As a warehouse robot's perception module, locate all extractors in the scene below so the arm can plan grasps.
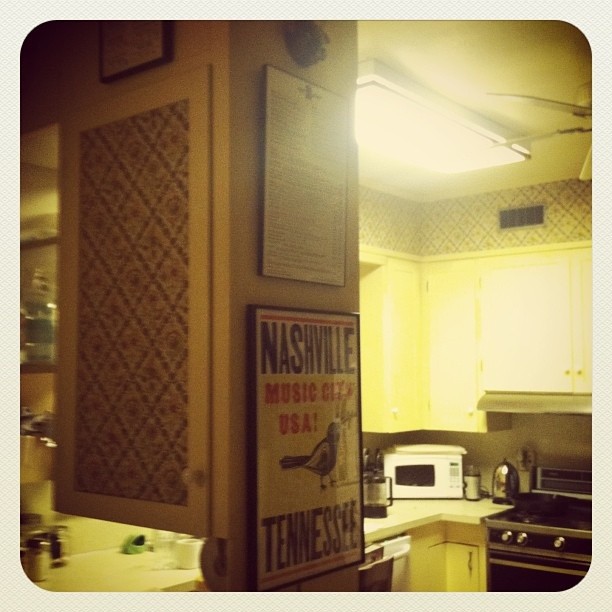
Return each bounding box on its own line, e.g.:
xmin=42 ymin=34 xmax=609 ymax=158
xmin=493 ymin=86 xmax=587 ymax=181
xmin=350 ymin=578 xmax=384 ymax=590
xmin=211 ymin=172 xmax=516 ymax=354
xmin=475 ymin=390 xmax=592 ymax=415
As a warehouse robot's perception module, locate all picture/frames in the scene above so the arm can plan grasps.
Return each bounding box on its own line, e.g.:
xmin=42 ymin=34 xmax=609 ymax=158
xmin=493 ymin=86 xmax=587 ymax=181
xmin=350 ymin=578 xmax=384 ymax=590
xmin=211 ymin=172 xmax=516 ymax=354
xmin=247 ymin=305 xmax=364 ymax=588
xmin=99 ymin=20 xmax=175 ymax=82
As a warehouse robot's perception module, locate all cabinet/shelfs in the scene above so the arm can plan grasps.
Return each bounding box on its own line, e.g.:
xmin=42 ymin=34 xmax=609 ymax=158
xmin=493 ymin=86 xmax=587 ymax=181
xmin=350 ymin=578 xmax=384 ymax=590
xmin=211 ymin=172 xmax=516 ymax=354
xmin=420 ymin=242 xmax=512 ymax=433
xmin=476 ymin=243 xmax=591 ymax=417
xmin=409 ymin=528 xmax=486 ymax=592
xmin=360 ymin=242 xmax=420 ymax=428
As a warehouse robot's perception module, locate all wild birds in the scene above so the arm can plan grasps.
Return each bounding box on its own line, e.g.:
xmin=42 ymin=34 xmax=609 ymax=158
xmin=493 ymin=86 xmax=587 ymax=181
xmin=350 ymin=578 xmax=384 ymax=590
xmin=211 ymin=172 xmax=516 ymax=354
xmin=280 ymin=422 xmax=343 ymax=490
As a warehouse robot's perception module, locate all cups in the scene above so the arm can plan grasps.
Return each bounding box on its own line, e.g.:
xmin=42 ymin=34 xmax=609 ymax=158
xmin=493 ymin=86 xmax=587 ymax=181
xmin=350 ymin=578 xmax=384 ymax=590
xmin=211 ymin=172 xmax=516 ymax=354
xmin=465 ymin=465 xmax=482 ymax=502
xmin=175 ymin=539 xmax=202 ymax=569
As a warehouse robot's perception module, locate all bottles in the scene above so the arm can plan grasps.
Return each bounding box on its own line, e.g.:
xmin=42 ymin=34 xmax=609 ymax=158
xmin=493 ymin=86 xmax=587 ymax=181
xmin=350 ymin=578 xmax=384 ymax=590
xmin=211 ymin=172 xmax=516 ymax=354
xmin=50 ymin=526 xmax=71 ymax=570
xmin=26 ymin=537 xmax=51 ymax=582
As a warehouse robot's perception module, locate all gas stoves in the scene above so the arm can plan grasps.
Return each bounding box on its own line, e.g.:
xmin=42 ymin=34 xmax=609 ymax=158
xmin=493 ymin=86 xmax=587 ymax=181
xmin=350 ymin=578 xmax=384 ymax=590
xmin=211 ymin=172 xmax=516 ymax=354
xmin=482 ymin=505 xmax=593 ymax=534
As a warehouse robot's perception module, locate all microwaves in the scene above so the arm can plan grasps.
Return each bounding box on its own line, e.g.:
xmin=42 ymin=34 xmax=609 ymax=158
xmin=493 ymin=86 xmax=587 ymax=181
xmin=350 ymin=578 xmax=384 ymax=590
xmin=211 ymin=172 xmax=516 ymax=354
xmin=383 ymin=452 xmax=462 ymax=501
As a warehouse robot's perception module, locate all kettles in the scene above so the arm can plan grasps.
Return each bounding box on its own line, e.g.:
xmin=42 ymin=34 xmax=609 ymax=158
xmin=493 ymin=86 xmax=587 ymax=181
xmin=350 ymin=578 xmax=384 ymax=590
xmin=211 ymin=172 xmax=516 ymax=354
xmin=363 ymin=467 xmax=392 ymax=518
xmin=491 ymin=459 xmax=519 ymax=505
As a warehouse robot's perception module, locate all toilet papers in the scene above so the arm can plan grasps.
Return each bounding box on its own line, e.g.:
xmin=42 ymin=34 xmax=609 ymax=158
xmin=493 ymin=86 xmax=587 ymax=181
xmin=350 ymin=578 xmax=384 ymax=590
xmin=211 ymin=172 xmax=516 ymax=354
xmin=174 ymin=536 xmax=203 ymax=572
